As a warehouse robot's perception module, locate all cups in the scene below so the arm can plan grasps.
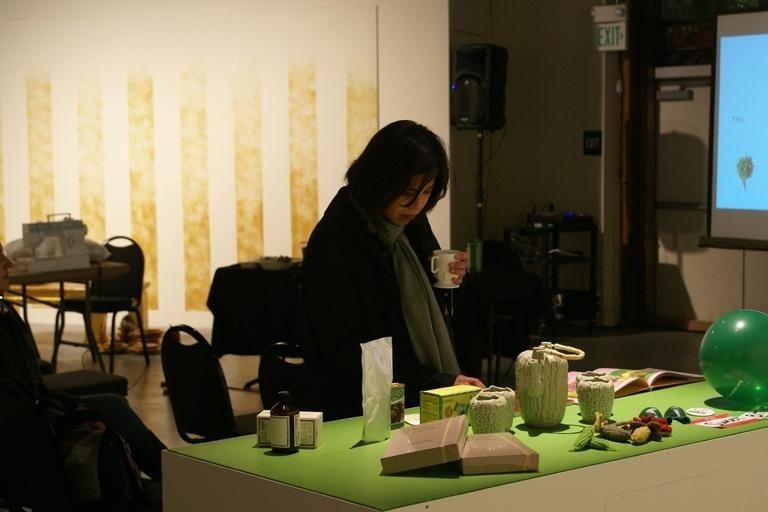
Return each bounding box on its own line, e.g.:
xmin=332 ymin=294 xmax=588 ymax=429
xmin=575 ymin=370 xmax=608 ymax=393
xmin=580 ymin=379 xmax=614 ymax=424
xmin=479 ymin=384 xmax=515 ymax=432
xmin=429 ymin=249 xmax=463 ymax=289
xmin=468 ymin=393 xmax=507 ymax=434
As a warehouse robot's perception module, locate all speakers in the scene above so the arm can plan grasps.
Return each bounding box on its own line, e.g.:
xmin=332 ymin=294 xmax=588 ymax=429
xmin=452 ymin=40 xmax=508 ymax=131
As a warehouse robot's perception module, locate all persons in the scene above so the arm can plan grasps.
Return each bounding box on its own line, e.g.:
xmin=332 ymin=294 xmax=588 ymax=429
xmin=1 ymin=246 xmax=165 ymax=510
xmin=299 ymin=119 xmax=486 ymax=416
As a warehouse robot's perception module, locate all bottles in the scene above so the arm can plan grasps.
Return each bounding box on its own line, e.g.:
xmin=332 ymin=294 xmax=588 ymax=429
xmin=466 ymin=239 xmax=483 ymax=272
xmin=271 ymin=389 xmax=301 ymax=455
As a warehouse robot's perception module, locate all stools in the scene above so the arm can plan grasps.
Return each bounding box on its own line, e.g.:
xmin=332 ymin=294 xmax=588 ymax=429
xmin=42 ymin=368 xmax=128 ymax=398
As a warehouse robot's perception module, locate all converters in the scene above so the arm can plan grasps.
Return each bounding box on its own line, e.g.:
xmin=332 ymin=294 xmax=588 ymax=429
xmin=548 ymin=202 xmax=554 ymax=212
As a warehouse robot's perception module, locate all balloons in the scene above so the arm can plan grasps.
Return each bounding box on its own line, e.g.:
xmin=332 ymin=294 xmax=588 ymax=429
xmin=699 ymin=308 xmax=768 ymax=408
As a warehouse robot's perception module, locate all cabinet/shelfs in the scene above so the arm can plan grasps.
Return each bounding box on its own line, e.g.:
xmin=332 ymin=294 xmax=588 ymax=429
xmin=508 ymin=215 xmax=597 ymax=338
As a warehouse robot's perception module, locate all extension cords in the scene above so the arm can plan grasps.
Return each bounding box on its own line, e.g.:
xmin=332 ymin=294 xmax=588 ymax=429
xmin=535 ymin=208 xmax=560 ymax=218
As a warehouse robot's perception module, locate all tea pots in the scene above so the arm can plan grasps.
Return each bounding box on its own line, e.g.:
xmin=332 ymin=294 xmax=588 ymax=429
xmin=515 ymin=342 xmax=586 ymax=428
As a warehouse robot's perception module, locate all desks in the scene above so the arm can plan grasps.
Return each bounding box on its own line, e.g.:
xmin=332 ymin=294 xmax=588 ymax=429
xmin=162 ymin=372 xmax=768 ymax=512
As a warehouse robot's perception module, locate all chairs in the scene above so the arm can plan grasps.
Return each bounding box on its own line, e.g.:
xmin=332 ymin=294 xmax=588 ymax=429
xmin=259 ymin=341 xmax=313 ymax=413
xmin=161 ymin=323 xmax=264 ymax=443
xmin=52 ymin=236 xmax=150 ymax=373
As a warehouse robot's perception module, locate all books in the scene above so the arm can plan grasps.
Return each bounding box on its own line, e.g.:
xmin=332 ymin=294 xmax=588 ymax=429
xmin=460 ymin=432 xmax=539 ymax=475
xmin=380 ymin=414 xmax=468 ymax=474
xmin=567 ymin=367 xmax=704 ymax=405
